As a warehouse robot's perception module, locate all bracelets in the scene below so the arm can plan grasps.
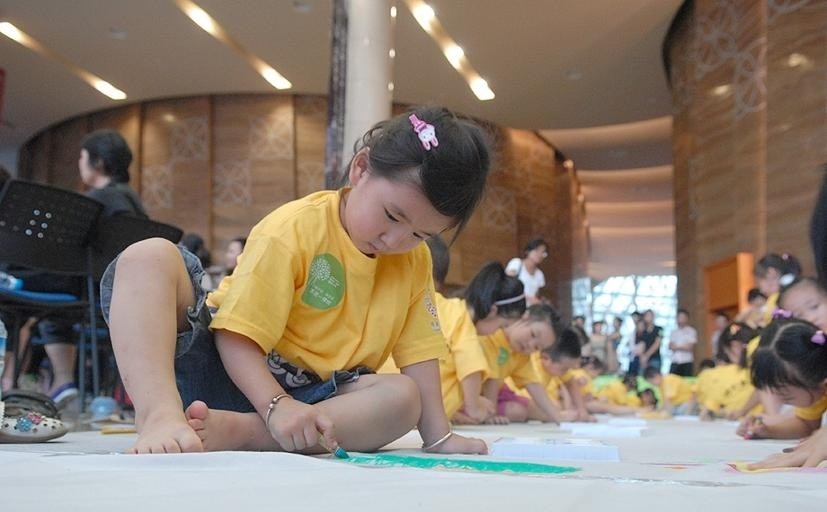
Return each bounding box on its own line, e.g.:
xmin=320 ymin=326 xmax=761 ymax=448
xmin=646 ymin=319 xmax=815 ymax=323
xmin=265 ymin=392 xmax=292 ymax=425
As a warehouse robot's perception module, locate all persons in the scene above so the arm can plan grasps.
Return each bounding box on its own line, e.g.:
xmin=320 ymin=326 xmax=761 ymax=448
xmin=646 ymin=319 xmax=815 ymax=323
xmin=99 ymin=103 xmax=492 ymax=456
xmin=180 ymin=231 xmax=244 ymax=291
xmin=691 ymin=168 xmax=827 ymax=471
xmin=36 ymin=128 xmax=151 ymax=419
xmin=380 ymin=231 xmax=698 ymax=425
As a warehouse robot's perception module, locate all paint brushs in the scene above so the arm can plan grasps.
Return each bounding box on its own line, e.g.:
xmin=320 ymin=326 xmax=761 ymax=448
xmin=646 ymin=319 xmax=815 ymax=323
xmin=743 ymin=419 xmax=762 ymax=439
xmin=318 ymin=434 xmax=350 ymax=458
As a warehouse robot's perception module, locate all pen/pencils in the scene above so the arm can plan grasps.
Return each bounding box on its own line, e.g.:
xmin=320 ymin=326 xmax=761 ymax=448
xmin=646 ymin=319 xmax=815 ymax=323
xmin=782 ymin=447 xmax=796 ymax=453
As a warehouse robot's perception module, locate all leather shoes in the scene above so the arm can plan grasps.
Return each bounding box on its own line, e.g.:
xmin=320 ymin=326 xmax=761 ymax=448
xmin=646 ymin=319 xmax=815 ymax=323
xmin=1 ymin=399 xmax=70 ymax=444
